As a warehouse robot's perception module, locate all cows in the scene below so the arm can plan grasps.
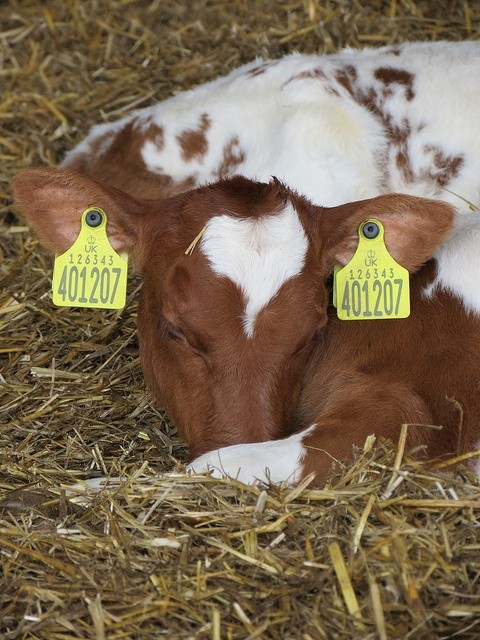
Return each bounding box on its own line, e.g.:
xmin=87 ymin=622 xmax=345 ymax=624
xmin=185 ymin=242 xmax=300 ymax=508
xmin=10 ymin=40 xmax=479 ymax=492
